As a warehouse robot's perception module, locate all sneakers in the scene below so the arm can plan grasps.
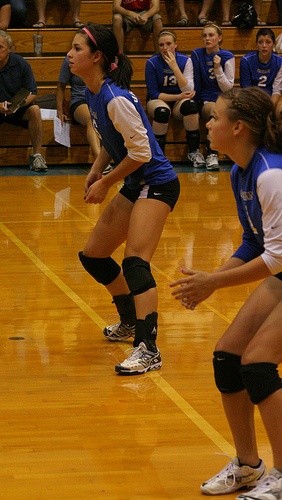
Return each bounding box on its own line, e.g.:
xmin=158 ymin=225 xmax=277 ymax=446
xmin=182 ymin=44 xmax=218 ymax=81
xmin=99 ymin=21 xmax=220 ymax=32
xmin=102 ymin=164 xmax=113 ymax=175
xmin=102 ymin=321 xmax=135 ymax=341
xmin=199 ymin=457 xmax=266 ymax=496
xmin=29 ymin=153 xmax=48 ymax=172
xmin=114 ymin=341 xmax=162 ymax=374
xmin=188 ymin=149 xmax=206 ymax=168
xmin=237 ymin=467 xmax=282 ymax=500
xmin=205 ymin=153 xmax=221 ymax=172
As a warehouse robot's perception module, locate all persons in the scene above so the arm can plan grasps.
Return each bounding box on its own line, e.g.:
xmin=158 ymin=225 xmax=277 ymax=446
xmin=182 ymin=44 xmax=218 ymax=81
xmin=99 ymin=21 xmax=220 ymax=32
xmin=113 ymin=0 xmax=164 ymax=55
xmin=191 ymin=23 xmax=235 ymax=170
xmin=173 ymin=0 xmax=267 ymax=26
xmin=0 ymin=0 xmax=85 ymax=28
xmin=170 ymin=85 xmax=282 ymax=500
xmin=145 ymin=28 xmax=207 ymax=169
xmin=0 ymin=30 xmax=49 ymax=172
xmin=56 ymin=55 xmax=113 ymax=175
xmin=67 ymin=23 xmax=180 ymax=375
xmin=239 ymin=29 xmax=282 ymax=98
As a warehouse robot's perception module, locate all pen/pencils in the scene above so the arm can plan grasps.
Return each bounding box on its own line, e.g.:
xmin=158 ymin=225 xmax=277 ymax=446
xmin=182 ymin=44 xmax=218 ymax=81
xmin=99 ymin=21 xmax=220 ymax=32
xmin=5 ymin=101 xmax=7 ymax=116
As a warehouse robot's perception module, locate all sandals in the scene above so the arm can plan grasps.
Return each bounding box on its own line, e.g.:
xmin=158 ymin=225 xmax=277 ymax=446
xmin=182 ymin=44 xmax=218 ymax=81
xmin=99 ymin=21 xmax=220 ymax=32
xmin=256 ymin=19 xmax=267 ymax=26
xmin=195 ymin=17 xmax=215 ymax=27
xmin=175 ymin=15 xmax=189 ymax=27
xmin=221 ymin=21 xmax=232 ymax=27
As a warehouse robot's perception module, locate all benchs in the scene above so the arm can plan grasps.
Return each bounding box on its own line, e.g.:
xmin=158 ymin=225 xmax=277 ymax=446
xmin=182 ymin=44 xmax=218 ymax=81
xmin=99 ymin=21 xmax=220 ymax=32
xmin=1 ymin=0 xmax=282 ymax=167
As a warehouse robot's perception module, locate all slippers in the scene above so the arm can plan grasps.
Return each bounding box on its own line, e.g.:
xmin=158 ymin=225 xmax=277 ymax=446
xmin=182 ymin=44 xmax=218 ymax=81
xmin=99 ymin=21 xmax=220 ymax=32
xmin=33 ymin=22 xmax=47 ymax=28
xmin=68 ymin=21 xmax=85 ymax=28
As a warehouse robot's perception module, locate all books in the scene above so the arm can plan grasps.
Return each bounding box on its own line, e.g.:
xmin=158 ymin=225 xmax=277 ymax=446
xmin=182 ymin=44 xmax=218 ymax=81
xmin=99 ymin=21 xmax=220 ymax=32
xmin=7 ymin=88 xmax=30 ymax=113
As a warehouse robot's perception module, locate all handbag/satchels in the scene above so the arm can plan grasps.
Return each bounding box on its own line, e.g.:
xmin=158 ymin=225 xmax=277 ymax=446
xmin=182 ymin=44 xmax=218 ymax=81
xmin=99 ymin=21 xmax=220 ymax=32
xmin=230 ymin=1 xmax=257 ymax=30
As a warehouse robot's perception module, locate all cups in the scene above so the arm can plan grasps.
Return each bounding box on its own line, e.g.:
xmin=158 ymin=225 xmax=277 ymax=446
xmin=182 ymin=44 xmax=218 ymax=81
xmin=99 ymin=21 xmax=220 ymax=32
xmin=33 ymin=34 xmax=44 ymax=56
